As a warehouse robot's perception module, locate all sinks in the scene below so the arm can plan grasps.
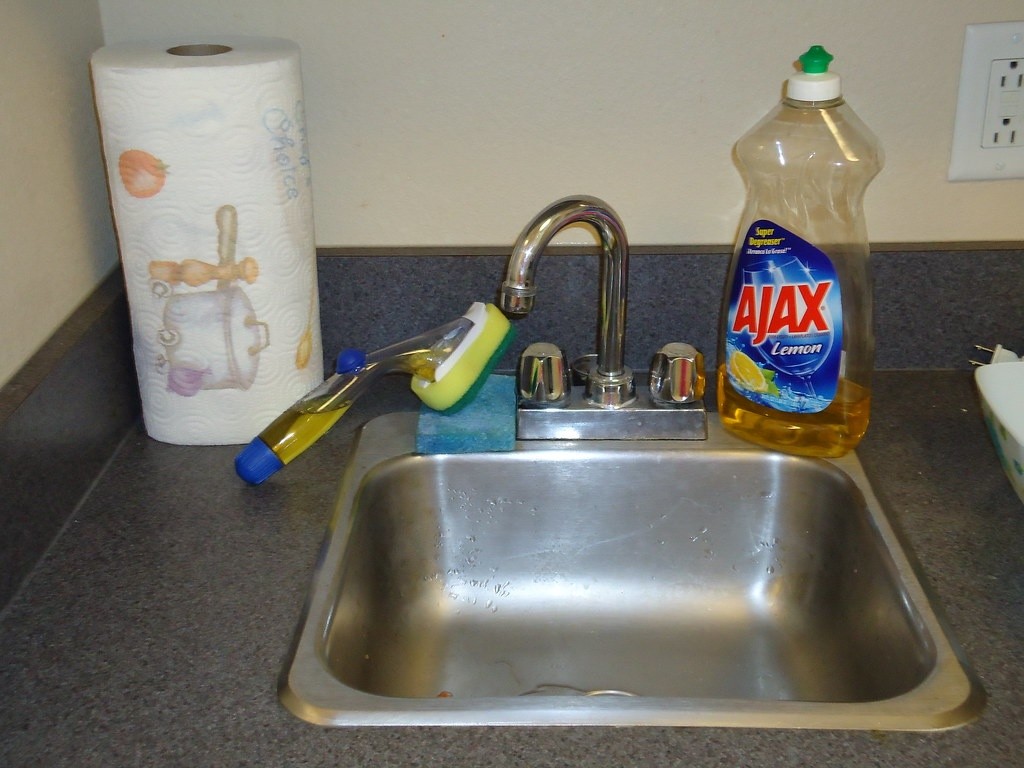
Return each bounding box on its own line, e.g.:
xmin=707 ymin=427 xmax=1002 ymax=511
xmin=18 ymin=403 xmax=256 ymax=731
xmin=277 ymin=407 xmax=989 ymax=733
xmin=499 ymin=192 xmax=709 ymax=441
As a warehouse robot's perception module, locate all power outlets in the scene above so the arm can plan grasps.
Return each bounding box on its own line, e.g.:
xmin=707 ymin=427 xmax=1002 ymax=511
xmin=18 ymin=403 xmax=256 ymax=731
xmin=948 ymin=20 xmax=1024 ymax=182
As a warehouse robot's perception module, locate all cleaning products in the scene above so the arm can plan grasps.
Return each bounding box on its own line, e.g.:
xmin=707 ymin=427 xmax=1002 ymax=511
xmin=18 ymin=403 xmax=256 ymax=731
xmin=715 ymin=42 xmax=888 ymax=460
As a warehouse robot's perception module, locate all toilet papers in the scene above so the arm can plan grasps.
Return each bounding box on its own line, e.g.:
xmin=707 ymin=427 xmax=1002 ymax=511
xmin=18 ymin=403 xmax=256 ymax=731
xmin=89 ymin=32 xmax=327 ymax=447
